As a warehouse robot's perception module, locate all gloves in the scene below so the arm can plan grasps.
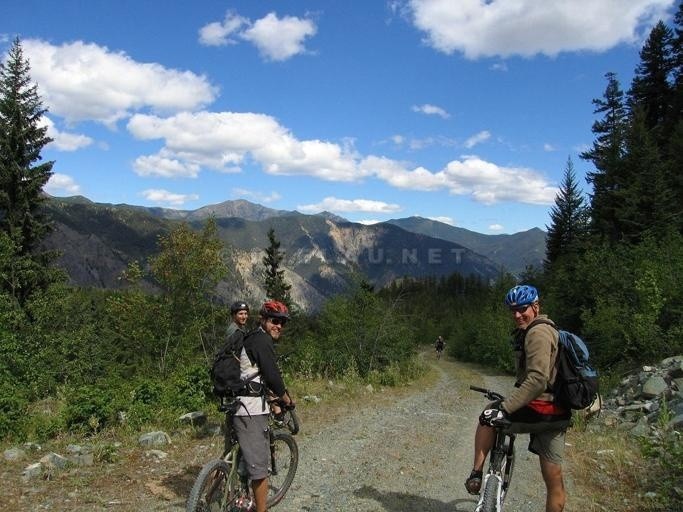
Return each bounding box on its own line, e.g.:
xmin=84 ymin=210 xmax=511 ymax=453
xmin=479 ymin=408 xmax=505 ymax=426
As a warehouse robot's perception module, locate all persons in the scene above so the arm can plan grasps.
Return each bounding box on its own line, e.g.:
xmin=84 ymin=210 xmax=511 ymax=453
xmin=224 ymin=301 xmax=249 ymax=344
xmin=434 ymin=336 xmax=446 ymax=360
xmin=232 ymin=301 xmax=296 ymax=512
xmin=466 ymin=285 xmax=572 ymax=511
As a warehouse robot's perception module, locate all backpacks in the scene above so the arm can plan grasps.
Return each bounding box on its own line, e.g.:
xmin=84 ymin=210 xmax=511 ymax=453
xmin=212 ymin=328 xmax=268 ymax=397
xmin=521 ymin=319 xmax=597 ymax=409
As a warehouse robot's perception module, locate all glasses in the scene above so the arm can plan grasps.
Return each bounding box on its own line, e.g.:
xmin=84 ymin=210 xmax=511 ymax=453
xmin=268 ymin=318 xmax=286 ymax=326
xmin=510 ymin=305 xmax=529 ymax=313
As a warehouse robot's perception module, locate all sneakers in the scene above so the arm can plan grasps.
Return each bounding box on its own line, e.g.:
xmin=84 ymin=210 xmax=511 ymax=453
xmin=467 ymin=469 xmax=482 ymax=493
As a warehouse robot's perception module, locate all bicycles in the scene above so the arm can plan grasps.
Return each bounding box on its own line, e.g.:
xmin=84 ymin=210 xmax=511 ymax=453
xmin=469 ymin=384 xmax=516 ymax=512
xmin=186 ymin=396 xmax=299 ymax=511
xmin=434 ymin=344 xmax=443 ymax=360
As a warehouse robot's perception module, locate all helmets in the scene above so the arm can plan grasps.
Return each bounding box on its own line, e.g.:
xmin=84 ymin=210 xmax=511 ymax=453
xmin=260 ymin=300 xmax=289 ymax=320
xmin=230 ymin=301 xmax=249 ymax=313
xmin=504 ymin=285 xmax=540 ymax=309
